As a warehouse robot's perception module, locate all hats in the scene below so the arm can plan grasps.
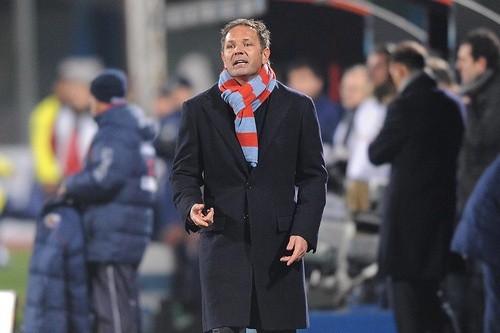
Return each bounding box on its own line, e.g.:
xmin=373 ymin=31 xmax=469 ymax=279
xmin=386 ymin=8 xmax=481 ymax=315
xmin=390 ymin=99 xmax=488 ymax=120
xmin=89 ymin=72 xmax=128 ymax=104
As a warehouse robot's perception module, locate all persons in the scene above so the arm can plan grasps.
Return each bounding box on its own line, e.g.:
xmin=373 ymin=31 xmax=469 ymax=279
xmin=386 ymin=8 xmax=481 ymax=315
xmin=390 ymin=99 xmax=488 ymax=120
xmin=285 ymin=28 xmax=500 ymax=333
xmin=170 ymin=19 xmax=329 ymax=333
xmin=28 ymin=56 xmax=202 ymax=333
